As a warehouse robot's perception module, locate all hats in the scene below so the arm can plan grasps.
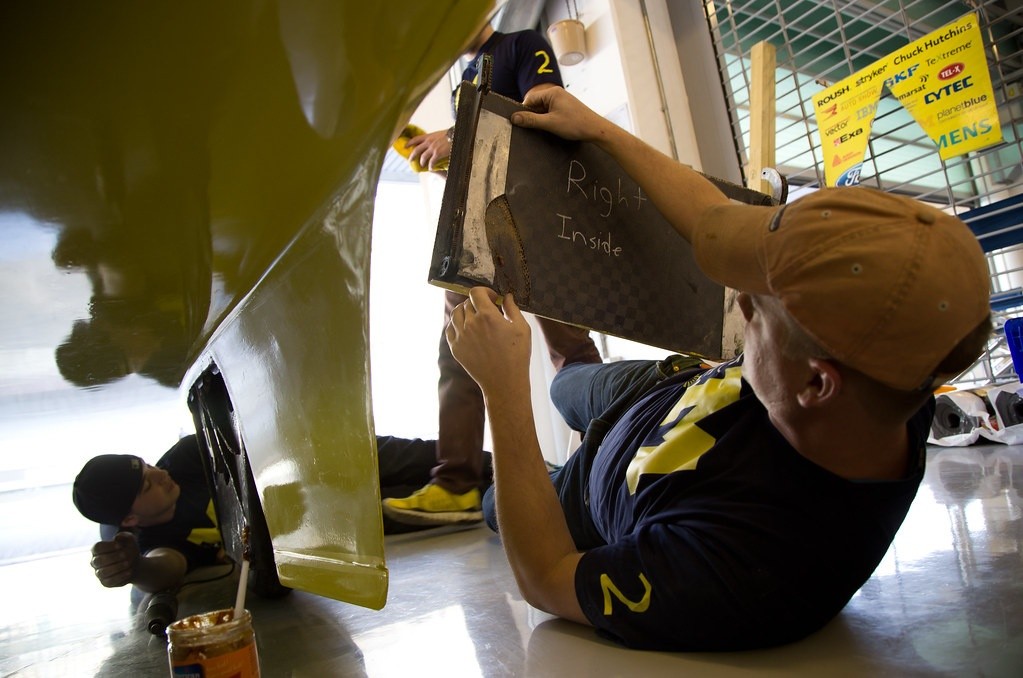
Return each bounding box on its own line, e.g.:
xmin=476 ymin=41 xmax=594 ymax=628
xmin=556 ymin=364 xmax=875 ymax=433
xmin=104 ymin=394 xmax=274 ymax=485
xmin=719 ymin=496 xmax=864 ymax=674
xmin=73 ymin=454 xmax=143 ymax=527
xmin=690 ymin=186 xmax=991 ymax=392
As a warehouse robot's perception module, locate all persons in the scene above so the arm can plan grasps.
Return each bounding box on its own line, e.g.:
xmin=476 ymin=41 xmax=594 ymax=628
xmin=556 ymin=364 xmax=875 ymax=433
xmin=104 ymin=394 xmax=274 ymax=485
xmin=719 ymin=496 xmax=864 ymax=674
xmin=445 ymin=83 xmax=993 ymax=652
xmin=381 ymin=20 xmax=603 ymax=528
xmin=70 ymin=430 xmax=565 ymax=595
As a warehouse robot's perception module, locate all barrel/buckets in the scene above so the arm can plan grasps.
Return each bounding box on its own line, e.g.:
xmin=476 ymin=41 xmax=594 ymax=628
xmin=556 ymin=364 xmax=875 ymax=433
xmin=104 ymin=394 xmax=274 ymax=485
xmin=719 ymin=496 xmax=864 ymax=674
xmin=547 ymin=20 xmax=586 ymax=66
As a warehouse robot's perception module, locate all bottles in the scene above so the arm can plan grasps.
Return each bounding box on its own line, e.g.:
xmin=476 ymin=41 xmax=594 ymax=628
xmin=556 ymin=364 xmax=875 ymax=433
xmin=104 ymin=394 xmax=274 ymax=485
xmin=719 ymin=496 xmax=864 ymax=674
xmin=165 ymin=609 xmax=261 ymax=678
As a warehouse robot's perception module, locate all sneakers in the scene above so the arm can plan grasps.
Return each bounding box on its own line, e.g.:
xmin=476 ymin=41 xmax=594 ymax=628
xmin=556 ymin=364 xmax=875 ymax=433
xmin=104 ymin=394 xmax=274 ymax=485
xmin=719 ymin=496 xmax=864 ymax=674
xmin=382 ymin=483 xmax=483 ymax=526
